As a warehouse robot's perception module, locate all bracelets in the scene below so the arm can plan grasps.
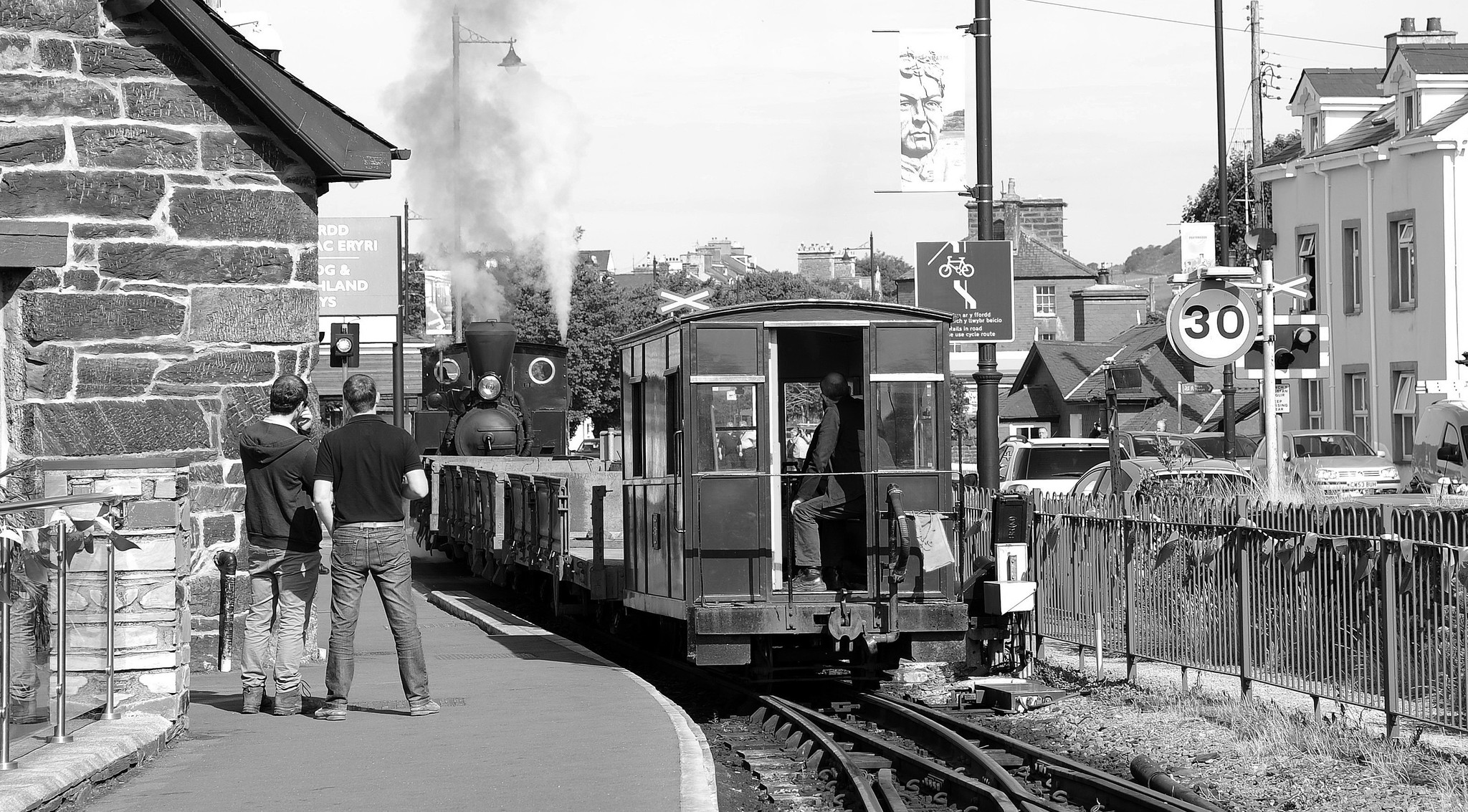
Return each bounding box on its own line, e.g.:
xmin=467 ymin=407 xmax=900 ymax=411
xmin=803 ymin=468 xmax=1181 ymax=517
xmin=739 ymin=451 xmax=742 ymax=453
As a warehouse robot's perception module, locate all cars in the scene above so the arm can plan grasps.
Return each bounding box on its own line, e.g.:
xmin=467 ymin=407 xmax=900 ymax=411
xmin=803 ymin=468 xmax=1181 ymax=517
xmin=997 ymin=430 xmax=1266 ymax=507
xmin=1250 ymin=428 xmax=1402 ymax=497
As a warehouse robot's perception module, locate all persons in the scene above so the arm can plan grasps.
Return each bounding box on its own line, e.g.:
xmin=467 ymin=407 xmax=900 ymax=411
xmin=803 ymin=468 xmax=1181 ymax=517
xmin=1038 ymin=427 xmax=1048 ymax=438
xmin=782 ymin=371 xmax=896 ymax=591
xmin=717 ymin=422 xmax=743 ymax=469
xmin=739 ymin=421 xmax=756 ymax=470
xmin=240 ymin=374 xmax=323 ymax=716
xmin=312 ymin=482 xmax=333 ymax=575
xmin=1088 ymin=423 xmax=1101 ymax=438
xmin=314 ymin=373 xmax=440 ymax=721
xmin=1325 ymin=437 xmax=1341 ymax=455
xmin=1156 ymin=421 xmax=1167 ymax=432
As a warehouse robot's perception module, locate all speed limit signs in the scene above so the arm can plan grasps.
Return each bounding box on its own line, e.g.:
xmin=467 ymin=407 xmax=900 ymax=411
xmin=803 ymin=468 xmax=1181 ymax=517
xmin=1169 ymin=283 xmax=1261 ymax=366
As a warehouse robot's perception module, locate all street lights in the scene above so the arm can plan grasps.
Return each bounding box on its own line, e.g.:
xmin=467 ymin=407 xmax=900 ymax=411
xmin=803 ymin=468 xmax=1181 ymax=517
xmin=407 ymin=290 xmax=978 ymax=692
xmin=447 ymin=5 xmax=529 ymax=347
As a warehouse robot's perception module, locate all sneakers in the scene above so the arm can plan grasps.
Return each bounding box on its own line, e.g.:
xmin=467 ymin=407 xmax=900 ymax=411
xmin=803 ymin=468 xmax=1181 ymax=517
xmin=818 ymin=565 xmax=845 ymax=590
xmin=779 ymin=568 xmax=827 ymax=591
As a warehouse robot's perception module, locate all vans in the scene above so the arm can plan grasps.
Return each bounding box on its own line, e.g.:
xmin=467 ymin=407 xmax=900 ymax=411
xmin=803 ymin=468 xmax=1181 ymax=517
xmin=1409 ymin=397 xmax=1468 ymax=498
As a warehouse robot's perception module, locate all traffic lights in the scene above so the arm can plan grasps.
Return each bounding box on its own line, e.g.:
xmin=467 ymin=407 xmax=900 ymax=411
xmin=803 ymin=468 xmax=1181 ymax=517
xmin=1247 ymin=324 xmax=1321 ymax=372
xmin=333 ymin=334 xmax=353 ymax=358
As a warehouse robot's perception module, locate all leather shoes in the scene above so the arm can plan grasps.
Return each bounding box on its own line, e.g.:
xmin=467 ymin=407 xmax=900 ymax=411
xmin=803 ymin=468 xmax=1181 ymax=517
xmin=318 ymin=563 xmax=329 ymax=574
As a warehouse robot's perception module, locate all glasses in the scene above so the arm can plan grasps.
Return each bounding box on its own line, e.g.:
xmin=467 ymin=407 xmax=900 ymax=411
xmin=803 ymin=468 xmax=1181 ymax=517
xmin=299 ymin=401 xmax=308 ymax=408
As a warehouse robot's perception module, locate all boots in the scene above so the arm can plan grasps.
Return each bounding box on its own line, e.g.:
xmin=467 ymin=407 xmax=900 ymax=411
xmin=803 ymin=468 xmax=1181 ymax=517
xmin=274 ymin=680 xmax=322 ymax=716
xmin=241 ymin=686 xmax=273 ymax=713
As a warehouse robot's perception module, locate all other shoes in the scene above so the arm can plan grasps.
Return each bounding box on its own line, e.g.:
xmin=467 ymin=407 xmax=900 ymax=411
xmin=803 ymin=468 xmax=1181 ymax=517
xmin=315 ymin=707 xmax=347 ymax=721
xmin=410 ymin=700 xmax=440 ymax=715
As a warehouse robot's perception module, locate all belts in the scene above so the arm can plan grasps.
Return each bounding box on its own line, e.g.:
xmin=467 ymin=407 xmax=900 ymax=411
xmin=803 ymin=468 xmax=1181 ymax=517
xmin=339 ymin=521 xmax=403 ymax=528
